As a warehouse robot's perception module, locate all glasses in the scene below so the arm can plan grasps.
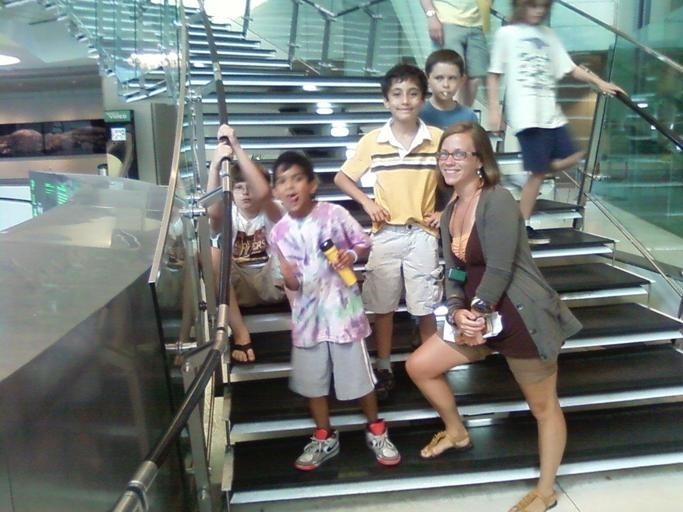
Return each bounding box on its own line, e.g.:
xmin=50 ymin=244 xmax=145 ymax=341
xmin=436 ymin=150 xmax=477 ymax=161
xmin=233 ymin=185 xmax=244 ymax=193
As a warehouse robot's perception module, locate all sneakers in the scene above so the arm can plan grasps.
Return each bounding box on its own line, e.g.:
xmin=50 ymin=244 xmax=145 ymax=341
xmin=294 ymin=430 xmax=340 ymax=470
xmin=527 ymin=225 xmax=550 ymax=245
xmin=374 ymin=369 xmax=397 ymax=401
xmin=365 ymin=418 xmax=401 ymax=465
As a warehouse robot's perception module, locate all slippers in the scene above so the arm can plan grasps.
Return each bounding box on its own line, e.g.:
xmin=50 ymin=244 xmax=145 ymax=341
xmin=231 ymin=342 xmax=257 ymax=364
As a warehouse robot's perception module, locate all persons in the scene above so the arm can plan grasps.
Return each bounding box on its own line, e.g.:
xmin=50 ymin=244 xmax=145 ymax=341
xmin=420 ymin=47 xmax=479 ymax=129
xmin=267 ymin=152 xmax=402 ymax=470
xmin=418 ymin=0 xmax=497 ymax=107
xmin=204 ymin=124 xmax=286 ymax=364
xmin=109 ymin=203 xmax=201 ymax=367
xmin=333 ymin=63 xmax=444 ymax=403
xmin=406 ymin=123 xmax=582 ymax=507
xmin=484 ymin=1 xmax=627 ymax=244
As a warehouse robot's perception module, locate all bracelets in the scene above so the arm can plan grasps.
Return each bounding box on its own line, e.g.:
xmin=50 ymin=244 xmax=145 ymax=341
xmin=345 ymin=248 xmax=359 ymax=261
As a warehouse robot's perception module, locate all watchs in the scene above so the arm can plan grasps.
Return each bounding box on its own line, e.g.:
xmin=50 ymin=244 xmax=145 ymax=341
xmin=423 ymin=8 xmax=438 ymax=17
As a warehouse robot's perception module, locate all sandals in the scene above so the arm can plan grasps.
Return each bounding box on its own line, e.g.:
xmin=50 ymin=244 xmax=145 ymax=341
xmin=420 ymin=431 xmax=474 ymax=460
xmin=508 ymin=491 xmax=558 ymax=512
